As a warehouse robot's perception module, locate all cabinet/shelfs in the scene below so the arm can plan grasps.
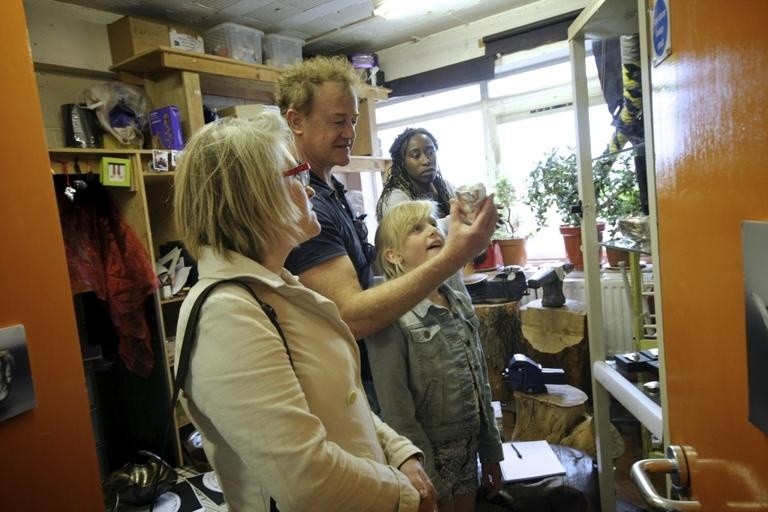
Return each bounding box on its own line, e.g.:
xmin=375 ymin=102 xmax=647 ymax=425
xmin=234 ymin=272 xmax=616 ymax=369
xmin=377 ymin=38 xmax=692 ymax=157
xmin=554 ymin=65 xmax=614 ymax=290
xmin=46 ymin=146 xmax=201 ymax=468
xmin=565 ymin=0 xmax=768 ymax=512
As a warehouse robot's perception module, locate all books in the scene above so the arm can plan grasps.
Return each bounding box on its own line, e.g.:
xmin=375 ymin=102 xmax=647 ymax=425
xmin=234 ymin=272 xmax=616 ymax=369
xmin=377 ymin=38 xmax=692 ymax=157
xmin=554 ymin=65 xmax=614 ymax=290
xmin=493 ymin=438 xmax=568 ymax=483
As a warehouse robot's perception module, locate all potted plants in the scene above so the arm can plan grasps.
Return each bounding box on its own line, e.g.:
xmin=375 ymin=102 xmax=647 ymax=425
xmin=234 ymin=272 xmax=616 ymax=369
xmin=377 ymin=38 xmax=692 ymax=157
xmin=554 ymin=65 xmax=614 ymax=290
xmin=523 ymin=147 xmax=644 ymax=273
xmin=493 ymin=177 xmax=522 ymax=266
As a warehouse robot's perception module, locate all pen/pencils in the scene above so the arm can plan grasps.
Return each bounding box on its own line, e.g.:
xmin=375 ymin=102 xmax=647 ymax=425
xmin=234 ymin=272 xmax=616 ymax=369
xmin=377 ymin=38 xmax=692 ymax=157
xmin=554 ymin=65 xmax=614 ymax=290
xmin=511 ymin=443 xmax=523 ymax=458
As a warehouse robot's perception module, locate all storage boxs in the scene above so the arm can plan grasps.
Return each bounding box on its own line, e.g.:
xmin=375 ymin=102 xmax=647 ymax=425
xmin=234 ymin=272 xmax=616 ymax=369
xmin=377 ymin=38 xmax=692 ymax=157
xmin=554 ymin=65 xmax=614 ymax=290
xmin=105 ymin=14 xmax=170 ymax=65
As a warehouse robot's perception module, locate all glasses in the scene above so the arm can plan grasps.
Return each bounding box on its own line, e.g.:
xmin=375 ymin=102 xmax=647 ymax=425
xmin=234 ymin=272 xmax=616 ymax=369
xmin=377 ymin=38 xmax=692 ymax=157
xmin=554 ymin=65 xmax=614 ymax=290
xmin=283 ymin=162 xmax=311 ymax=187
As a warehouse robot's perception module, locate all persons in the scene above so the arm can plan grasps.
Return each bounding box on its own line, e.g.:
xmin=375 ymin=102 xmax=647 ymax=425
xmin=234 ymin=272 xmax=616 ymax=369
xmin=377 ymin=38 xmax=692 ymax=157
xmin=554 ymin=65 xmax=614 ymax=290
xmin=364 ymin=195 xmax=507 ymax=510
xmin=260 ymin=53 xmax=501 ymax=385
xmin=163 ymin=107 xmax=448 ymax=512
xmin=373 ymin=126 xmax=476 ymax=300
